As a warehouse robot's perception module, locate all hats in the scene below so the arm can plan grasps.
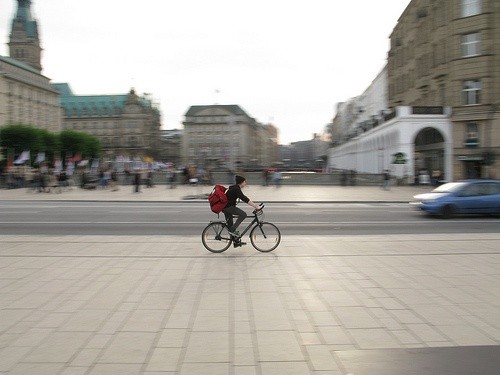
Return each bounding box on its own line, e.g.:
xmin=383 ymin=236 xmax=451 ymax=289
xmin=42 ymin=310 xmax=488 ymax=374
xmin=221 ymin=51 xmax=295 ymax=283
xmin=236 ymin=176 xmax=246 ymax=185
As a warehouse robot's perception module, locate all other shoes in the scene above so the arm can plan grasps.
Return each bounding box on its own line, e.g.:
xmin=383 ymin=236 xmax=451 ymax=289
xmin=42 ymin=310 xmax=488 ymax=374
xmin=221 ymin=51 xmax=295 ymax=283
xmin=229 ymin=230 xmax=242 ymax=239
xmin=234 ymin=241 xmax=247 ymax=248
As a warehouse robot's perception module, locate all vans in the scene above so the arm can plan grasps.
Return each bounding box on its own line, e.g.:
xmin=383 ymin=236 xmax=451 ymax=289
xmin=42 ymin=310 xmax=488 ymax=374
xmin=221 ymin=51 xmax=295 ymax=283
xmin=409 ymin=179 xmax=500 ymax=217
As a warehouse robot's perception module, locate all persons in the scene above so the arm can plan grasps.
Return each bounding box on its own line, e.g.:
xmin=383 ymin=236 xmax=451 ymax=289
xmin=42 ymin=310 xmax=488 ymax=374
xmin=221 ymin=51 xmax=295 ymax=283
xmin=263 ymin=169 xmax=441 ymax=191
xmin=222 ymin=176 xmax=261 ymax=248
xmin=4 ymin=167 xmax=236 ymax=193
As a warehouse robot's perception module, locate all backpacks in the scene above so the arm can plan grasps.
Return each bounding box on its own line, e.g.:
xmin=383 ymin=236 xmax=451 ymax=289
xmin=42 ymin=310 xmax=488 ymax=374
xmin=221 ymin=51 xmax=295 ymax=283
xmin=209 ymin=185 xmax=230 ymax=219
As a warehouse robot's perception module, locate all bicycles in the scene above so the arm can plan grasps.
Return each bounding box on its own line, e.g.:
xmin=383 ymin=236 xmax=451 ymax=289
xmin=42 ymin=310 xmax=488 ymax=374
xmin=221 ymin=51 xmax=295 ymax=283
xmin=201 ymin=202 xmax=281 ymax=253
